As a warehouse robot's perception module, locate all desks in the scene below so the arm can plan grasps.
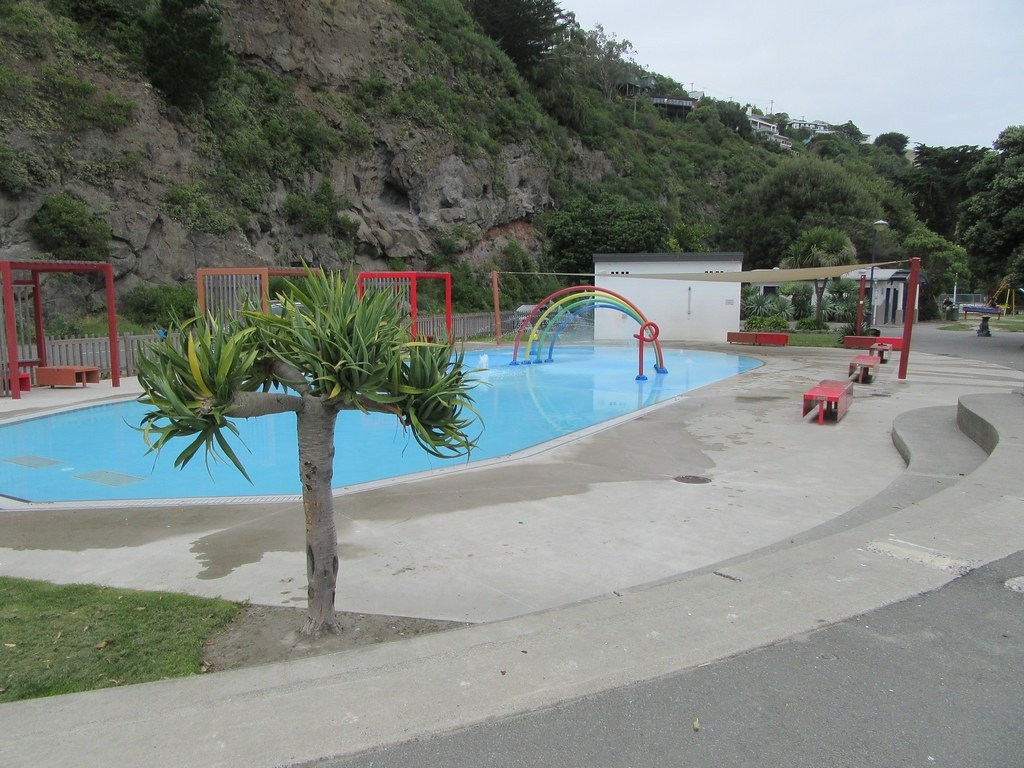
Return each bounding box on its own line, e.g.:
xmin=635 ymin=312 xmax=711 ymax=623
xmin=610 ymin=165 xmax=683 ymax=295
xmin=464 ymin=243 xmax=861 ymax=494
xmin=0 ymin=360 xmax=44 ymax=386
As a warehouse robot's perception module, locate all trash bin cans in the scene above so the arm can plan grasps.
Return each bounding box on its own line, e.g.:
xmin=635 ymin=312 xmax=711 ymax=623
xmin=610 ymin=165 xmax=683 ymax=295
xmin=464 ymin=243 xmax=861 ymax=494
xmin=946 ymin=305 xmax=959 ymax=320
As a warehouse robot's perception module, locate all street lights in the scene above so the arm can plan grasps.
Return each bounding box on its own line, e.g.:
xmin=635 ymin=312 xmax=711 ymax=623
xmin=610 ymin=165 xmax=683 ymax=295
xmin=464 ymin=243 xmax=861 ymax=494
xmin=865 ymin=219 xmax=889 ymax=331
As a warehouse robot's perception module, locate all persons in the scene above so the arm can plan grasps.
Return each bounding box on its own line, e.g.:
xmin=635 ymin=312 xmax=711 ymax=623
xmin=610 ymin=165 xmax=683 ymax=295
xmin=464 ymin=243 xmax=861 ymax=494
xmin=942 ymin=297 xmax=953 ymax=313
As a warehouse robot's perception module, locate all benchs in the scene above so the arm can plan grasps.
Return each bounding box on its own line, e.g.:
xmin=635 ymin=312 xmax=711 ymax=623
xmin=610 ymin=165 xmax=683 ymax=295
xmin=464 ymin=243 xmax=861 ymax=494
xmin=3 ymin=375 xmax=32 ymax=392
xmin=801 ymin=379 xmax=855 ymax=425
xmin=727 ymin=331 xmax=789 ymax=346
xmin=843 ymin=336 xmax=904 ymax=352
xmin=847 ymin=354 xmax=881 ymax=385
xmin=35 ymin=364 xmax=100 ymax=389
